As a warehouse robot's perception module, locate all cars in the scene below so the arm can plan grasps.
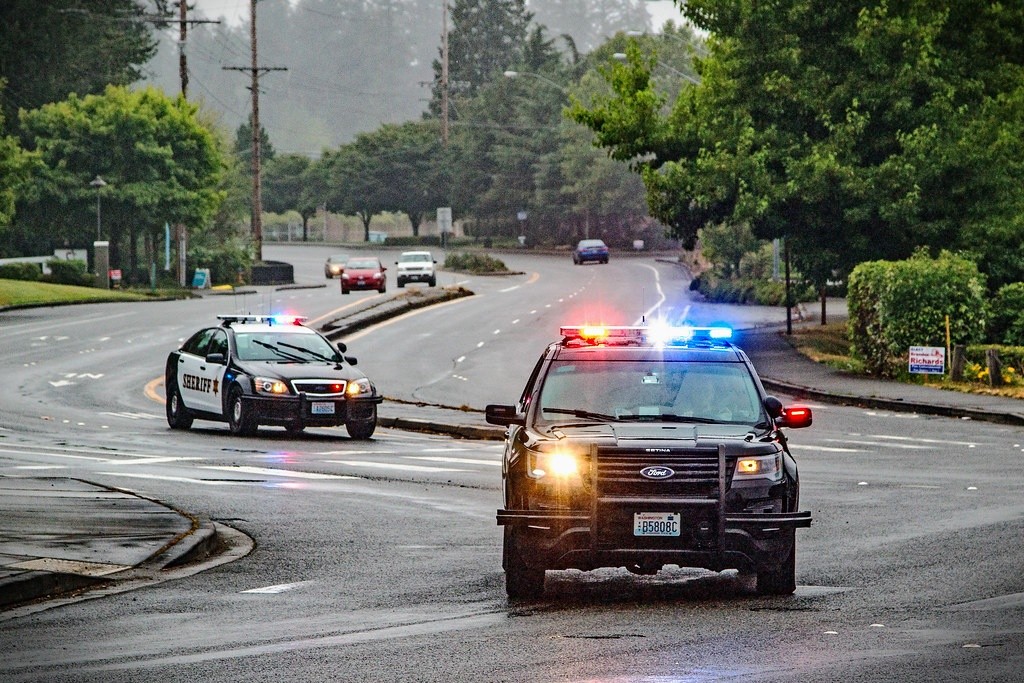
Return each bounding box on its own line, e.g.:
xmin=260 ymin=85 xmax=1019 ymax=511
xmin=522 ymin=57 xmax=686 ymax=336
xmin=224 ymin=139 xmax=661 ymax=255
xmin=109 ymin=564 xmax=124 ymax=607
xmin=339 ymin=258 xmax=387 ymax=294
xmin=325 ymin=254 xmax=349 ymax=279
xmin=165 ymin=312 xmax=383 ymax=440
xmin=572 ymin=239 xmax=609 ymax=265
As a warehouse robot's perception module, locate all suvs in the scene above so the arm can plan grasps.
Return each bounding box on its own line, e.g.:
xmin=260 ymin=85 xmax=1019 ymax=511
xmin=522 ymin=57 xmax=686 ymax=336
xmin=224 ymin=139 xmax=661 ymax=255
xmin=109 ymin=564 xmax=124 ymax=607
xmin=395 ymin=251 xmax=437 ymax=287
xmin=485 ymin=324 xmax=813 ymax=596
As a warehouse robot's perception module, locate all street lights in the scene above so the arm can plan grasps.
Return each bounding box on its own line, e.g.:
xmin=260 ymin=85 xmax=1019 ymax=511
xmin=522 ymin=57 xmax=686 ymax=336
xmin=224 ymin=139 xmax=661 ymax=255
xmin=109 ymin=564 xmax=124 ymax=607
xmin=90 ymin=174 xmax=108 ymax=242
xmin=626 ymin=31 xmax=780 ymax=282
